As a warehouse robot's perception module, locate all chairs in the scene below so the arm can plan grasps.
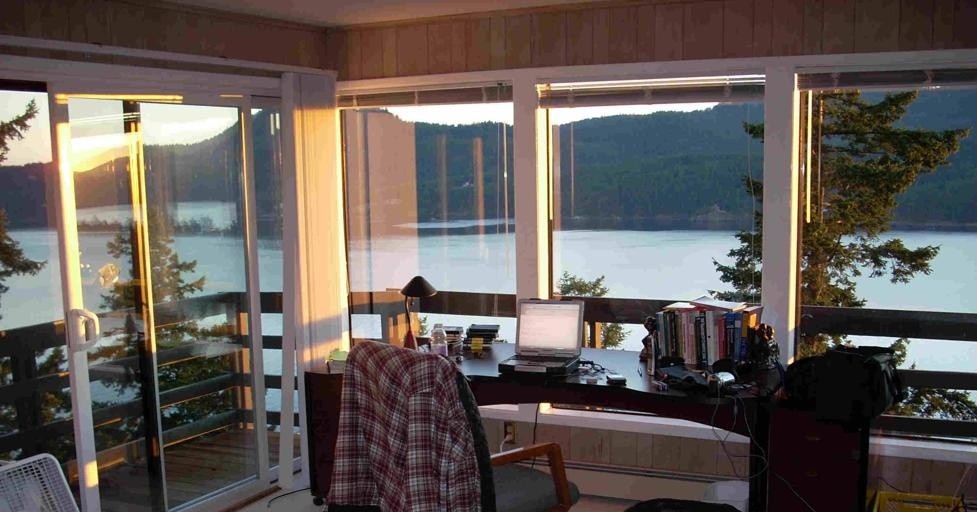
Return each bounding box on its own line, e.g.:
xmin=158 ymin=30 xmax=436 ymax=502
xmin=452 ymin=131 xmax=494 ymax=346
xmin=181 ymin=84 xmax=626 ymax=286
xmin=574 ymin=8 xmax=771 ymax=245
xmin=320 ymin=341 xmax=571 ymax=512
xmin=613 ymin=348 xmax=897 ymax=511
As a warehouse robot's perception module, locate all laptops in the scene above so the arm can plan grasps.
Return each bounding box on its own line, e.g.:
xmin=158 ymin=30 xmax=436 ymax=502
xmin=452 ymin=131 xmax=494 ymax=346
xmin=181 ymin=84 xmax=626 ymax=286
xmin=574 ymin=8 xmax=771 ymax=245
xmin=497 ymin=297 xmax=585 ymax=379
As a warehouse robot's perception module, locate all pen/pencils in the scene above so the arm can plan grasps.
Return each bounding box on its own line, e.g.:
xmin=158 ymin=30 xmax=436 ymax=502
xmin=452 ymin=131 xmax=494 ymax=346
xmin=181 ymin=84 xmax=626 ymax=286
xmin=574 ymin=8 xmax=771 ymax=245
xmin=638 ymin=368 xmax=642 ymax=376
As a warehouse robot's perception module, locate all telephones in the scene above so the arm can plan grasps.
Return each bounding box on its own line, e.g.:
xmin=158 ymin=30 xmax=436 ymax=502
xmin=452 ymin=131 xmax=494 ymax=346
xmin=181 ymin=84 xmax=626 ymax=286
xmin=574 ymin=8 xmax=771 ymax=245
xmin=647 ymin=330 xmax=659 ymax=376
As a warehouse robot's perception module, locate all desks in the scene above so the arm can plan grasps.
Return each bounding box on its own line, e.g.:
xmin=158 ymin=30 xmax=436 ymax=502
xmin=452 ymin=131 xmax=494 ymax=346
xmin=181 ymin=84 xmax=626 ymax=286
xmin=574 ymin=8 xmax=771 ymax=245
xmin=293 ymin=343 xmax=976 ymax=512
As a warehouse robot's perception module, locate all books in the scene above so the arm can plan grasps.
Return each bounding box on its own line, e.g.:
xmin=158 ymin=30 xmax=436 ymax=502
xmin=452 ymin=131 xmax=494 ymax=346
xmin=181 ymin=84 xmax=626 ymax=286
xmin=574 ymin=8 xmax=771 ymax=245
xmin=662 ymin=302 xmax=703 ymax=311
xmin=441 ymin=326 xmax=500 ymax=350
xmin=690 ymin=299 xmax=747 ymax=312
xmin=647 ymin=306 xmax=764 ymax=375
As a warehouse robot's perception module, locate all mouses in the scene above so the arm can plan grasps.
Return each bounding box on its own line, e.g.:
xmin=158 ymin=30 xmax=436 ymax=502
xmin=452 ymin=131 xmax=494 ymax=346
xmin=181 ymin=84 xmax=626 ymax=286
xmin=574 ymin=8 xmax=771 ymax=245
xmin=605 ymin=372 xmax=626 ymax=385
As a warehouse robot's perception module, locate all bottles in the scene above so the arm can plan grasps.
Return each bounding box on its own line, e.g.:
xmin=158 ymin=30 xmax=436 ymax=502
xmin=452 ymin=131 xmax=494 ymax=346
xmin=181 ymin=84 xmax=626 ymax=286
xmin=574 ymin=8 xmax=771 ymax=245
xmin=430 ymin=324 xmax=449 ymax=359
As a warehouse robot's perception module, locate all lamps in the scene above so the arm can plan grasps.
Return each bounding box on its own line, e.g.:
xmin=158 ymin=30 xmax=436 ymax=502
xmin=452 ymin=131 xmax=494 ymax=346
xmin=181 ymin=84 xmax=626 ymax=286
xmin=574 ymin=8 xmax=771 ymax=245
xmin=398 ymin=275 xmax=439 ymax=351
